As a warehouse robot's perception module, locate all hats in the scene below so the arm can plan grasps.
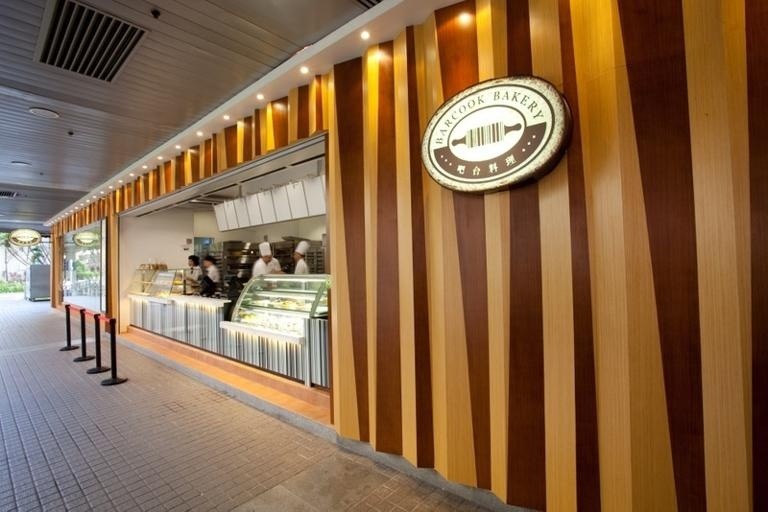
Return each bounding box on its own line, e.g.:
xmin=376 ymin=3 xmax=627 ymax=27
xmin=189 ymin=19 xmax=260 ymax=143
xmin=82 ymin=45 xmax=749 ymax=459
xmin=259 ymin=242 xmax=271 ymax=256
xmin=205 ymin=256 xmax=216 ymax=262
xmin=295 ymin=241 xmax=310 ymax=255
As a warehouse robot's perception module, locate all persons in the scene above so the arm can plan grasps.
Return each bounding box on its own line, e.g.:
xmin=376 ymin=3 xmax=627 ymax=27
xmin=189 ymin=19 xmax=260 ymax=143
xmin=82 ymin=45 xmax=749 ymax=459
xmin=183 ymin=255 xmax=203 ymax=295
xmin=251 ymin=241 xmax=281 ymax=279
xmin=293 ymin=240 xmax=310 ymax=276
xmin=202 ymin=254 xmax=220 ymax=297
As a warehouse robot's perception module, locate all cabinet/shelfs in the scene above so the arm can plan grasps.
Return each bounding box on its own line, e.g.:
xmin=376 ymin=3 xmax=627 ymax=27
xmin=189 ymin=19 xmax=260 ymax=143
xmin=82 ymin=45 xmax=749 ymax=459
xmin=25 ymin=265 xmax=50 ymax=302
xmin=127 ymin=233 xmax=330 ymax=391
